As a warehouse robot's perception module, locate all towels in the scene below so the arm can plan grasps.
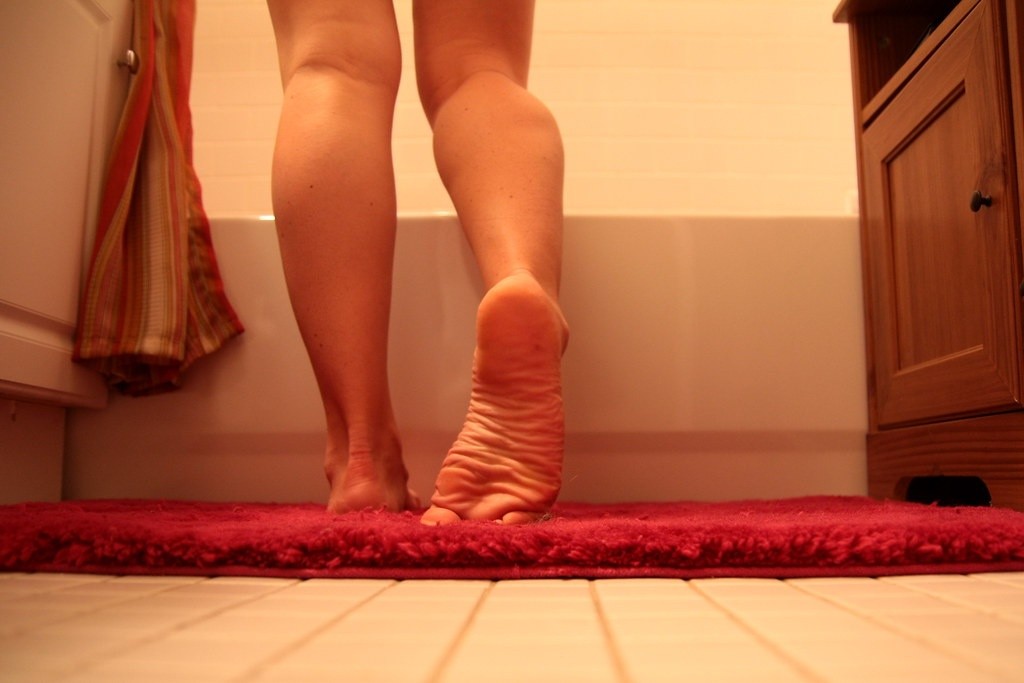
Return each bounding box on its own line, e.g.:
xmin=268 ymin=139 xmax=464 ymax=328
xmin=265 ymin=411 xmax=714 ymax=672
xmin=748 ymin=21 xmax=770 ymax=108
xmin=69 ymin=1 xmax=247 ymax=400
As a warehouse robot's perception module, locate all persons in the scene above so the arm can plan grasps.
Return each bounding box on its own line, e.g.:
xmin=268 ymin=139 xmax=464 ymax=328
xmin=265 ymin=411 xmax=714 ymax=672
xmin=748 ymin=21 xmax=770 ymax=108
xmin=267 ymin=0 xmax=570 ymax=526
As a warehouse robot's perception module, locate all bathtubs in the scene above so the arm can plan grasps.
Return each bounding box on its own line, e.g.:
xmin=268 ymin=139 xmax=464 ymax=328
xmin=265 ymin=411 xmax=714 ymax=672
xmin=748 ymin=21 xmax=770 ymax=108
xmin=58 ymin=212 xmax=865 ymax=505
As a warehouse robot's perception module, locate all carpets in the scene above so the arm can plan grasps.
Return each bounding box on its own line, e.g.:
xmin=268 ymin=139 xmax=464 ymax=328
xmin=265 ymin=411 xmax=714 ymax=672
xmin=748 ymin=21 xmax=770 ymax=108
xmin=0 ymin=496 xmax=1024 ymax=574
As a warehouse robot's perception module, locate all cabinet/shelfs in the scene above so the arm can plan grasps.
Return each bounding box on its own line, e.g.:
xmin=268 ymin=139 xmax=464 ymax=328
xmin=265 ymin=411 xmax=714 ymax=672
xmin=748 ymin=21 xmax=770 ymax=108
xmin=0 ymin=0 xmax=134 ymax=413
xmin=833 ymin=0 xmax=1024 ymax=510
